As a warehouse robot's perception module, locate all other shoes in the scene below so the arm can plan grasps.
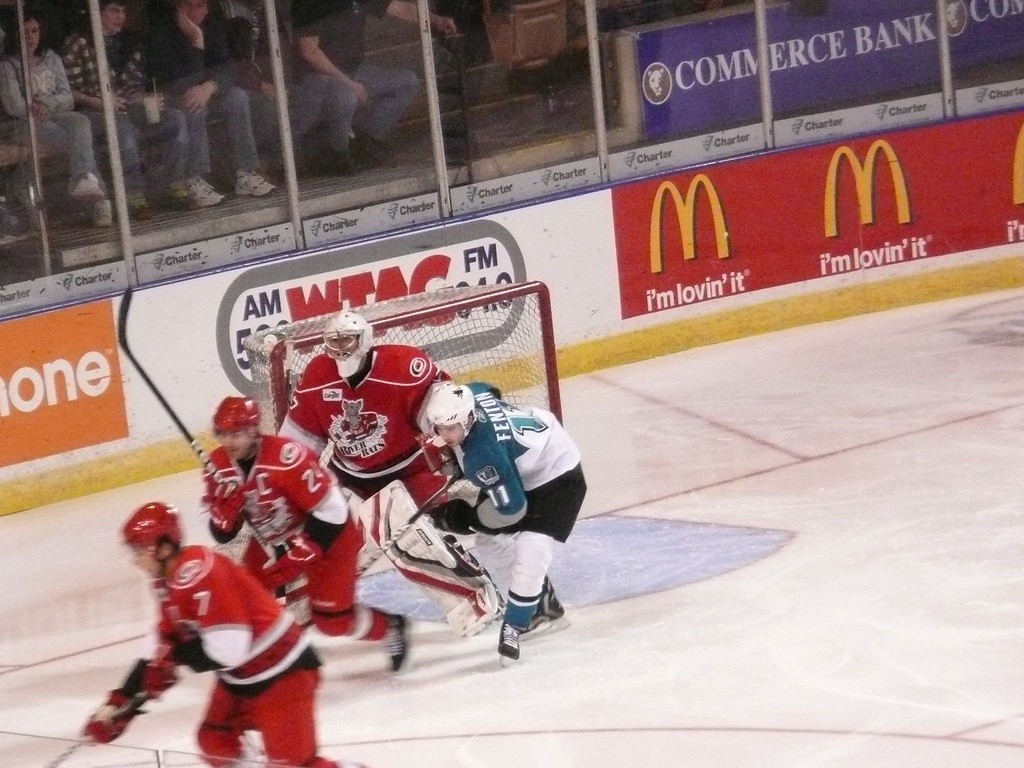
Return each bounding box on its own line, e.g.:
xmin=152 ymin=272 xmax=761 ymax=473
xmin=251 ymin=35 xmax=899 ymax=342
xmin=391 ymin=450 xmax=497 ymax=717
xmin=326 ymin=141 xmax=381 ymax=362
xmin=109 ymin=194 xmax=153 ymax=221
xmin=143 ymin=179 xmax=191 ymax=211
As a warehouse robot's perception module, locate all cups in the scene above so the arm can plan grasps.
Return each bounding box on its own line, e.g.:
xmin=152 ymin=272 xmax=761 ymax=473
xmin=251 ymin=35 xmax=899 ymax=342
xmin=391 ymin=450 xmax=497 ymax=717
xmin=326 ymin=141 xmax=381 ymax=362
xmin=144 ymin=97 xmax=160 ymax=125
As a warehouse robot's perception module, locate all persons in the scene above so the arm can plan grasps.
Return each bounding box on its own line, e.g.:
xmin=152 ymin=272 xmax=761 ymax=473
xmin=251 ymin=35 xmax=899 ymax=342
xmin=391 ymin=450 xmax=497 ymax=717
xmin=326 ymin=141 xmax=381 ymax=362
xmin=435 ymin=0 xmax=503 ymax=162
xmin=418 ymin=381 xmax=589 ymax=666
xmin=197 ymin=397 xmax=409 ymax=676
xmin=275 ymin=311 xmax=504 ymax=639
xmin=218 ymin=0 xmax=322 ymax=183
xmin=291 ymin=0 xmax=458 ymax=176
xmin=541 ymin=0 xmax=626 ymax=132
xmin=86 ymin=502 xmax=367 ymax=768
xmin=56 ymin=0 xmax=199 ymax=219
xmin=0 ymin=14 xmax=112 ymax=227
xmin=145 ymin=0 xmax=278 ymax=207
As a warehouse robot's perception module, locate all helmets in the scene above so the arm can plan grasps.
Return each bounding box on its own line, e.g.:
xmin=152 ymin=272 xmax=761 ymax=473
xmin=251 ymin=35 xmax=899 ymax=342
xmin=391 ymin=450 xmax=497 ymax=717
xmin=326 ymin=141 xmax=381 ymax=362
xmin=321 ymin=311 xmax=375 ymax=377
xmin=424 ymin=382 xmax=477 ymax=438
xmin=121 ymin=502 xmax=182 ymax=551
xmin=212 ymin=396 xmax=262 ymax=435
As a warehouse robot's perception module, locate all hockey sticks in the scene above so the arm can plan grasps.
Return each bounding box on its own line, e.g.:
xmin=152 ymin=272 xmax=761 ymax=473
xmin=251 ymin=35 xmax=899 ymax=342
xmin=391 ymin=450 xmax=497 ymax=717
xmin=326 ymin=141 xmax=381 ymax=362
xmin=354 ymin=473 xmax=461 ymax=579
xmin=116 ymin=287 xmax=274 ymax=557
xmin=45 ymin=658 xmax=149 ymax=768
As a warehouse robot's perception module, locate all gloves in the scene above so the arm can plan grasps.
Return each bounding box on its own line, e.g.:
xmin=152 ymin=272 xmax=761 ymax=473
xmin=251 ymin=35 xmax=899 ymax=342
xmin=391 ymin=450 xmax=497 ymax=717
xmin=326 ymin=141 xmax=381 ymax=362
xmin=81 ymin=688 xmax=146 ymax=744
xmin=208 ymin=479 xmax=258 ymax=533
xmin=426 ymin=498 xmax=477 ymax=536
xmin=262 ymin=532 xmax=324 ymax=591
xmin=145 ymin=658 xmax=179 ymax=699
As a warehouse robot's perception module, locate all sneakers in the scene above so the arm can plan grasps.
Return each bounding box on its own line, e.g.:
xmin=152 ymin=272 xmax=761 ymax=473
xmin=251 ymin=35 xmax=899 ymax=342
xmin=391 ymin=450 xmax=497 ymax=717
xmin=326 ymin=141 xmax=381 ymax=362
xmin=383 ymin=613 xmax=410 ymax=672
xmin=91 ymin=199 xmax=112 ymax=228
xmin=517 ymin=575 xmax=571 ymax=642
xmin=496 ymin=621 xmax=521 ymax=666
xmin=170 ymin=173 xmax=228 ymax=209
xmin=66 ymin=172 xmax=106 ymax=201
xmin=234 ymin=167 xmax=277 ymax=198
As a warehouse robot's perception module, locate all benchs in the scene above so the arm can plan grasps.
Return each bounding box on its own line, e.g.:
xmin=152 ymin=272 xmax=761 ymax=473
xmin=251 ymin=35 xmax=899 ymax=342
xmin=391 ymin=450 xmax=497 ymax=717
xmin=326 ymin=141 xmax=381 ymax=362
xmin=0 ymin=14 xmax=547 ymax=261
